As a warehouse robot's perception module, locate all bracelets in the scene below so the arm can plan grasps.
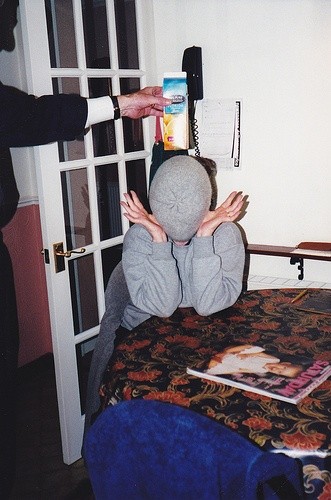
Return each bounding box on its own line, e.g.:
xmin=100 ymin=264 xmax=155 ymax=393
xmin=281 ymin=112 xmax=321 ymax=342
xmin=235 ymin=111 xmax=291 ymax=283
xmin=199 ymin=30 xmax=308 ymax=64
xmin=109 ymin=95 xmax=121 ymax=120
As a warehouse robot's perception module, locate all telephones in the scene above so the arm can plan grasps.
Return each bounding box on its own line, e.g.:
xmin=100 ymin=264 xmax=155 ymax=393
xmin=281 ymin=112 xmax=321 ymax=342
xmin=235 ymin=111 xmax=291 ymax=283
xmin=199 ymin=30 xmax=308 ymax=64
xmin=182 ymin=46 xmax=203 ymax=101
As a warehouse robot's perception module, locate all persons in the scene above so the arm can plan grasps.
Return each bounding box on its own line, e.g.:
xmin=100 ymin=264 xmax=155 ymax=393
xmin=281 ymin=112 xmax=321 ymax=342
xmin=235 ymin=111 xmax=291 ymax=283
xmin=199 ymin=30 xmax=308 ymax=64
xmin=204 ymin=344 xmax=305 ymax=379
xmin=1 ymin=0 xmax=172 ymax=500
xmin=112 ymin=152 xmax=247 ymax=346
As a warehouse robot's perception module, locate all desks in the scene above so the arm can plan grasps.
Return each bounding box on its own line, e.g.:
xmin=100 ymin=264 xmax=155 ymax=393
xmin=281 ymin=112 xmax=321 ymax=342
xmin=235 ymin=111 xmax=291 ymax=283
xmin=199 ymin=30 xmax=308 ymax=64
xmin=100 ymin=289 xmax=331 ymax=500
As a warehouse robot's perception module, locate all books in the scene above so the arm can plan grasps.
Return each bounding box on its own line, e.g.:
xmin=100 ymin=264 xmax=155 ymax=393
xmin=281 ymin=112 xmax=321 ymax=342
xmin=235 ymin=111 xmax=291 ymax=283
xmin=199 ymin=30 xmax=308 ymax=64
xmin=186 ymin=339 xmax=331 ymax=404
